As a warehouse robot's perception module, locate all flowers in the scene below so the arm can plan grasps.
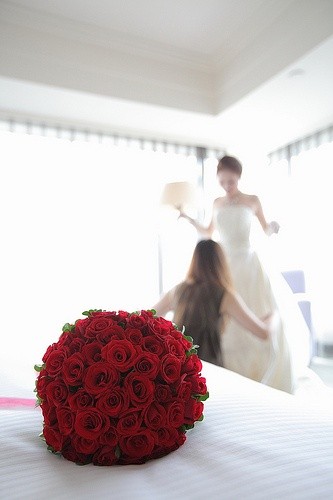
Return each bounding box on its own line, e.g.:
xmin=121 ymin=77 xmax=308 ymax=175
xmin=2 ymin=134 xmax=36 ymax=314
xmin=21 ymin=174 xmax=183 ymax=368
xmin=35 ymin=309 xmax=209 ymax=474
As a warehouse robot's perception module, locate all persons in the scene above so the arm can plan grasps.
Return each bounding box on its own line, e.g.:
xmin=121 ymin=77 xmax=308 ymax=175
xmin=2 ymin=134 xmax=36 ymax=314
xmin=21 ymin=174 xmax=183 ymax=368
xmin=174 ymin=153 xmax=284 ymax=381
xmin=146 ymin=238 xmax=273 ymax=374
